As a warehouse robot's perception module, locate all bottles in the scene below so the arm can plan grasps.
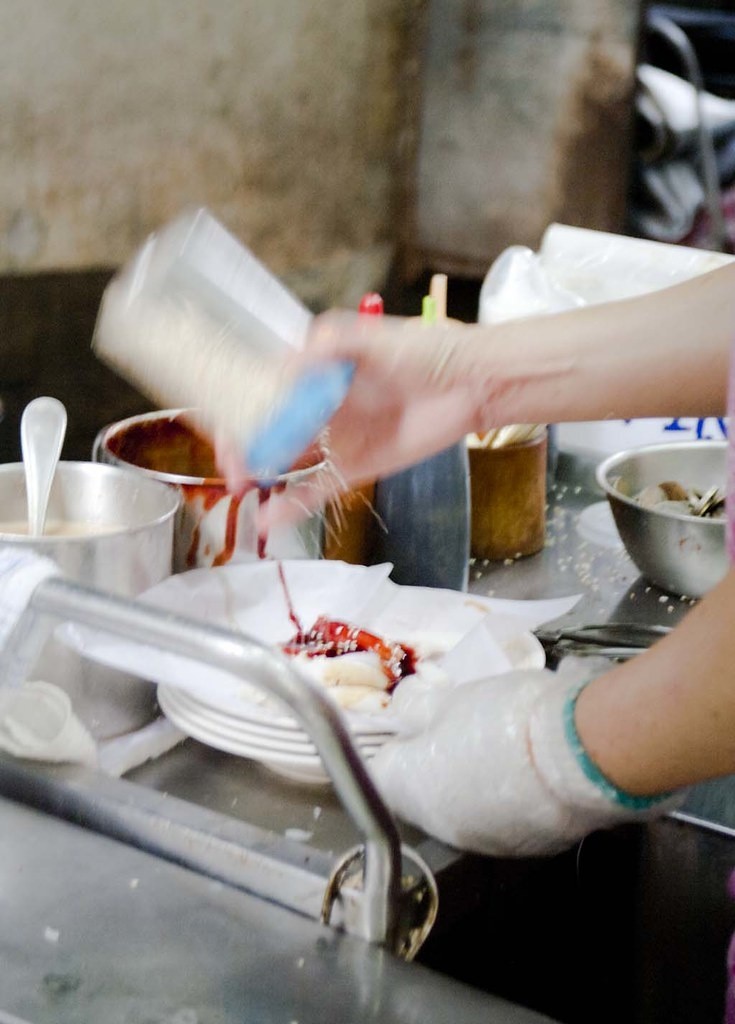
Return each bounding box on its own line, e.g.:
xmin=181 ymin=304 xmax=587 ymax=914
xmin=379 ymin=438 xmax=469 ymax=592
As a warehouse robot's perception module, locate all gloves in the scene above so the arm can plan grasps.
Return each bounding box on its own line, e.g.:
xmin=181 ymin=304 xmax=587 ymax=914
xmin=367 ymin=656 xmax=689 ymax=856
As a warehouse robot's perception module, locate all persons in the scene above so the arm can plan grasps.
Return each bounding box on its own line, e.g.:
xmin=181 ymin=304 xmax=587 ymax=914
xmin=214 ymin=263 xmax=735 ymax=1024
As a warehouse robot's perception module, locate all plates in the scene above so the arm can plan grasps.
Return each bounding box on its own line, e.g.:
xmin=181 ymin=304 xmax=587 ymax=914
xmin=52 ymin=556 xmax=584 ymax=786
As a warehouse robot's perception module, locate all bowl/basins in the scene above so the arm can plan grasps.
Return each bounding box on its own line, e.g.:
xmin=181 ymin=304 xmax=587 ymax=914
xmin=596 ymin=438 xmax=732 ymax=595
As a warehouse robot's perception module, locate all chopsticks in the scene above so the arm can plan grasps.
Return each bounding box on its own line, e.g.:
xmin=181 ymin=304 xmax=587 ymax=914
xmin=467 ymin=421 xmax=547 ymax=450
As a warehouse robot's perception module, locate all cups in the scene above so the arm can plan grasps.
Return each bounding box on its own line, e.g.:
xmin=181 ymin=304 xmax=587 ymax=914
xmin=0 ymin=460 xmax=180 ymax=745
xmin=467 ymin=426 xmax=547 ymax=557
xmin=100 ymin=405 xmax=329 ymax=576
